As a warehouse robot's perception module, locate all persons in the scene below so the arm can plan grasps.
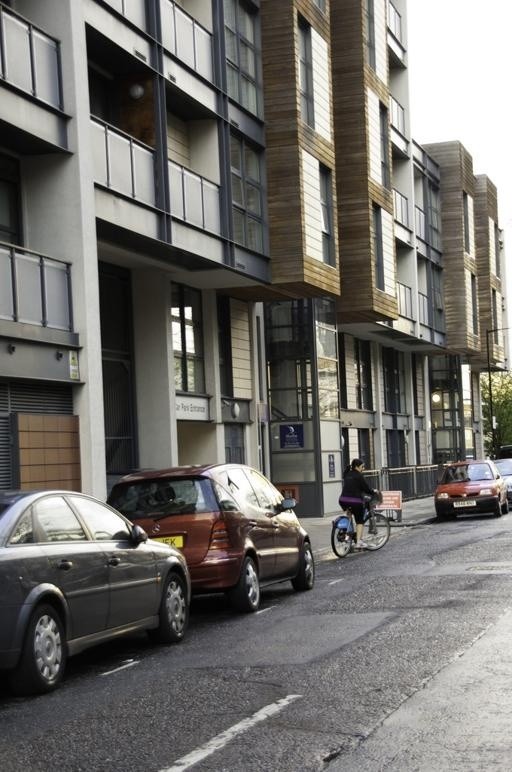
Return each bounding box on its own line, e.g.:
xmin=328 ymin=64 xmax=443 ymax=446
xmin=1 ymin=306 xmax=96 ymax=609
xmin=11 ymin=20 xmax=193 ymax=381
xmin=337 ymin=458 xmax=379 ymax=550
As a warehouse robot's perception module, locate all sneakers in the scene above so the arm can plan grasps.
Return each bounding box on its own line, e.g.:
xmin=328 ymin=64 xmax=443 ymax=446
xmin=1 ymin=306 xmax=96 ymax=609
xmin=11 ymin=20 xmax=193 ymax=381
xmin=354 ymin=540 xmax=368 ymax=550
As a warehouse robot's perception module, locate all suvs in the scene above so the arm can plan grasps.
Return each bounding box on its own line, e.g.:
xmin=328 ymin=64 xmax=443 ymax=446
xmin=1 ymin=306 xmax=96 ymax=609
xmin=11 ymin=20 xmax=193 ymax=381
xmin=103 ymin=460 xmax=318 ymax=616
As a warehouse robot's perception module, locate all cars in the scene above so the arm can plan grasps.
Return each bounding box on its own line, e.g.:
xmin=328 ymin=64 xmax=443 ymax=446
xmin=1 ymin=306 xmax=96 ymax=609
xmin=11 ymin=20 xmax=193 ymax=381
xmin=1 ymin=486 xmax=193 ymax=698
xmin=434 ymin=460 xmax=511 ymax=523
xmin=492 ymin=458 xmax=512 ymax=508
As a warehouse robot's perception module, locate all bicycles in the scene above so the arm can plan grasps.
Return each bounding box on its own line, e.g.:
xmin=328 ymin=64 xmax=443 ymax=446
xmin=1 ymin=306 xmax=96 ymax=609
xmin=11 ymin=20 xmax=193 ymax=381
xmin=330 ymin=493 xmax=392 ymax=560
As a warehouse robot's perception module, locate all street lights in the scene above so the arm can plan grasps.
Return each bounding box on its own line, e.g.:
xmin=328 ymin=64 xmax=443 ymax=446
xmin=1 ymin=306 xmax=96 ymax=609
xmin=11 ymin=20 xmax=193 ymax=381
xmin=485 ymin=326 xmax=510 ymax=460
